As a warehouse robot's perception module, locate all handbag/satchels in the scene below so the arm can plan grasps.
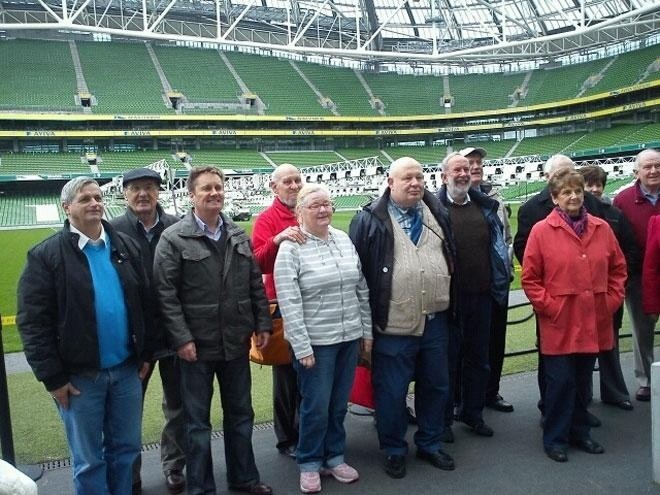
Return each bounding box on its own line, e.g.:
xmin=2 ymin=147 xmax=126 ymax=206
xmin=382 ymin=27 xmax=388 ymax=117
xmin=248 ymin=300 xmax=293 ymax=366
xmin=348 ymin=365 xmax=375 ymax=410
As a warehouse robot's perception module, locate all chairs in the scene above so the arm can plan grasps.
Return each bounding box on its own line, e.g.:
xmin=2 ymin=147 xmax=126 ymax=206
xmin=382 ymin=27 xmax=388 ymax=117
xmin=526 ymin=45 xmax=659 ymax=202
xmin=0 ymin=39 xmax=266 ymax=228
xmin=258 ymin=56 xmax=527 ymax=213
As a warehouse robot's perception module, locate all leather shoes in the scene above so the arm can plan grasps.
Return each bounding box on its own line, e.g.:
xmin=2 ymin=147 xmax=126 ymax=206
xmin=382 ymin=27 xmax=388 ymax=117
xmin=382 ymin=454 xmax=407 ymax=479
xmin=166 ymin=472 xmax=186 ymax=493
xmin=635 ymin=385 xmax=652 ymax=402
xmin=567 ymin=433 xmax=604 ymax=455
xmin=280 ymin=446 xmax=297 ymax=458
xmin=227 ymin=479 xmax=274 ymax=494
xmin=587 ymin=410 xmax=602 ymax=428
xmin=490 ymin=391 xmax=514 ymax=413
xmin=458 ymin=413 xmax=495 ymax=438
xmin=542 ymin=442 xmax=568 ymax=462
xmin=414 ymin=445 xmax=456 ymax=471
xmin=440 ymin=424 xmax=455 ymax=443
xmin=610 ymin=399 xmax=634 ymax=410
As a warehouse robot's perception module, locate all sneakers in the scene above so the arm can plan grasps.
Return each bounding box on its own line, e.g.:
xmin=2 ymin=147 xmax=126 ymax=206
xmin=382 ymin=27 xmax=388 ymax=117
xmin=318 ymin=462 xmax=359 ymax=484
xmin=298 ymin=471 xmax=322 ymax=493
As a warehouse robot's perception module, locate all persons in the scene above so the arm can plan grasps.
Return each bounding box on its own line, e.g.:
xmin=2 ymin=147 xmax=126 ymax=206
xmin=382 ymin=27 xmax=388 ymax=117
xmin=15 ymin=176 xmax=158 ymax=495
xmin=273 ymin=182 xmax=374 ymax=492
xmin=641 ymin=214 xmax=660 ymax=320
xmin=579 ymin=165 xmax=634 ymax=412
xmin=611 ymin=148 xmax=660 ymax=401
xmin=436 ymin=152 xmax=511 ymax=443
xmin=348 ymin=156 xmax=463 ymax=479
xmin=152 ymin=166 xmax=273 ymax=495
xmin=453 ymin=146 xmax=514 ymax=422
xmin=520 ymin=166 xmax=629 ymax=462
xmin=250 ymin=162 xmax=308 ymax=461
xmin=103 ymin=168 xmax=186 ymax=493
xmin=512 ymin=153 xmax=606 ymax=428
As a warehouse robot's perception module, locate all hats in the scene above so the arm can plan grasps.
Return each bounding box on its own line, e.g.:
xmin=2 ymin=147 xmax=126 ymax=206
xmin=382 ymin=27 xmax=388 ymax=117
xmin=122 ymin=167 xmax=163 ymax=188
xmin=459 ymin=146 xmax=487 ymax=159
